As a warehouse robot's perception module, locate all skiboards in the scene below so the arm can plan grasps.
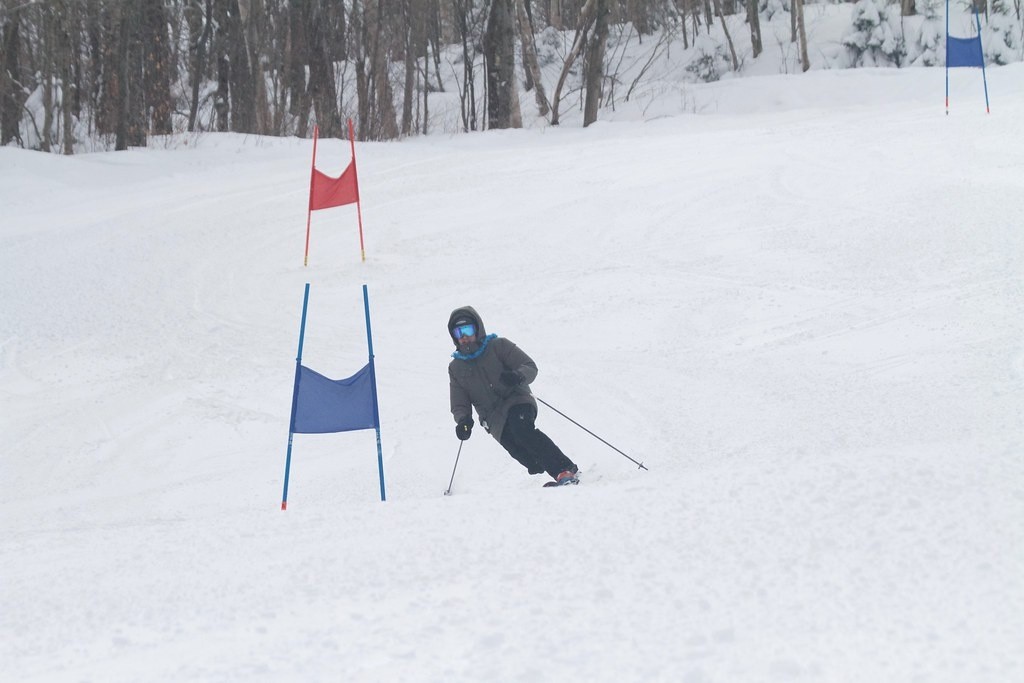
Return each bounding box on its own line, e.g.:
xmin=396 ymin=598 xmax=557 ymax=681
xmin=542 ymin=481 xmax=597 ymax=487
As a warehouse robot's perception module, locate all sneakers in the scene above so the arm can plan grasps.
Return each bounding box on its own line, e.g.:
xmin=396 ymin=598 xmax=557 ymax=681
xmin=557 ymin=464 xmax=580 ymax=485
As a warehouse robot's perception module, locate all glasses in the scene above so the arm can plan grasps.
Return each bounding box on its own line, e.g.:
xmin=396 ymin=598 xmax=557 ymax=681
xmin=450 ymin=324 xmax=476 ymax=340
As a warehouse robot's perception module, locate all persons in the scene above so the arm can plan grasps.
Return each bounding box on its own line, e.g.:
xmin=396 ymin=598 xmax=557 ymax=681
xmin=448 ymin=306 xmax=582 ymax=486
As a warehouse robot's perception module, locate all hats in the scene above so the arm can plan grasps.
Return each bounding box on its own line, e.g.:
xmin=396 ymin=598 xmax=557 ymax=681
xmin=448 ymin=310 xmax=477 ymax=330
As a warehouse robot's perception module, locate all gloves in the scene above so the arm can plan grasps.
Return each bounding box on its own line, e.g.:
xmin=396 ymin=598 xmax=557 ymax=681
xmin=456 ymin=419 xmax=475 ymax=440
xmin=499 ymin=372 xmax=521 ymax=387
